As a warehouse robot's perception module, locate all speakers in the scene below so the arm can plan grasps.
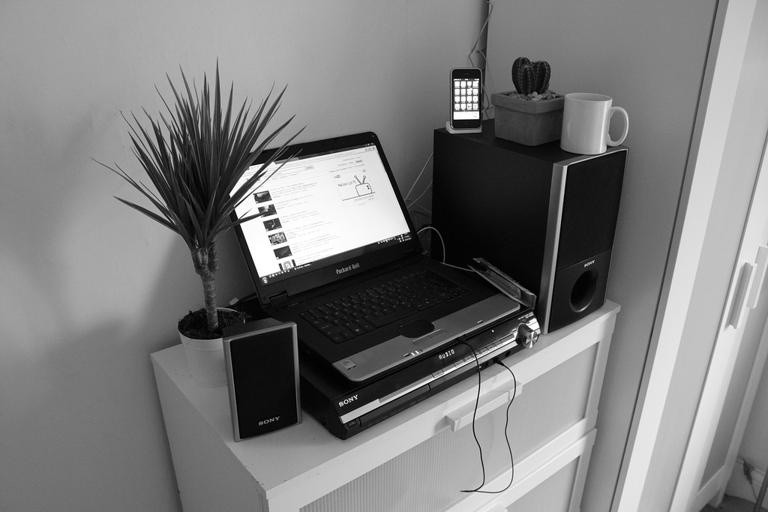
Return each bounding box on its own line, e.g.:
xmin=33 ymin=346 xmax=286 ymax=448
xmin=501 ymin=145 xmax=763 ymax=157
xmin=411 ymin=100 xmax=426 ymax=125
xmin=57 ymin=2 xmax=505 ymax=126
xmin=220 ymin=316 xmax=304 ymax=442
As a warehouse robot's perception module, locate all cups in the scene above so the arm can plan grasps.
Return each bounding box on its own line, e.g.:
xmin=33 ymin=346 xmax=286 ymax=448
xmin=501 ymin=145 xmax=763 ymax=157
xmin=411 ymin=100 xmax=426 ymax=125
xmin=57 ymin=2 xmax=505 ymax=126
xmin=559 ymin=91 xmax=630 ymax=156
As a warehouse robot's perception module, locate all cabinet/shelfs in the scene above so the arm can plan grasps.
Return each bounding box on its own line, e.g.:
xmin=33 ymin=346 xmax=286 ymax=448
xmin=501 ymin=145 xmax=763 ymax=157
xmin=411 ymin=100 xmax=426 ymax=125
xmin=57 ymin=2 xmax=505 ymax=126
xmin=147 ymin=298 xmax=623 ymax=512
xmin=482 ymin=0 xmax=768 ymax=512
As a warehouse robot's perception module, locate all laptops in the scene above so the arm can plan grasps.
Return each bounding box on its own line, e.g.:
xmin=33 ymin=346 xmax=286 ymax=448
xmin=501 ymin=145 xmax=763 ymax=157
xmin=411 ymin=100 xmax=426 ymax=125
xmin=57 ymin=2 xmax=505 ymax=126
xmin=221 ymin=130 xmax=522 ymax=386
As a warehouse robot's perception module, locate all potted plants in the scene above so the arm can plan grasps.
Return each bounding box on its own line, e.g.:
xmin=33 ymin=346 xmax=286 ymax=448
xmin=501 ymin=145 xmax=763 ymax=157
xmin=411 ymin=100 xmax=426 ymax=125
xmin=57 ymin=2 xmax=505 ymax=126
xmin=88 ymin=55 xmax=312 ymax=389
xmin=490 ymin=57 xmax=565 ymax=146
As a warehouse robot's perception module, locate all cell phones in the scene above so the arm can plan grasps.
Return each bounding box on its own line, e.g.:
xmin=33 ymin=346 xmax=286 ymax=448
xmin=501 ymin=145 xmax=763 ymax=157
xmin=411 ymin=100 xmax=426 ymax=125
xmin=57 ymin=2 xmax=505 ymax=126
xmin=450 ymin=67 xmax=483 ymax=129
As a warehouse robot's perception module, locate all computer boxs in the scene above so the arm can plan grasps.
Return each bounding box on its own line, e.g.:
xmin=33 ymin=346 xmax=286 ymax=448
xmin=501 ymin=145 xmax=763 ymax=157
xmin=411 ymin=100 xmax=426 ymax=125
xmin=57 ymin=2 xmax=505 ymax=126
xmin=430 ymin=117 xmax=629 ymax=336
